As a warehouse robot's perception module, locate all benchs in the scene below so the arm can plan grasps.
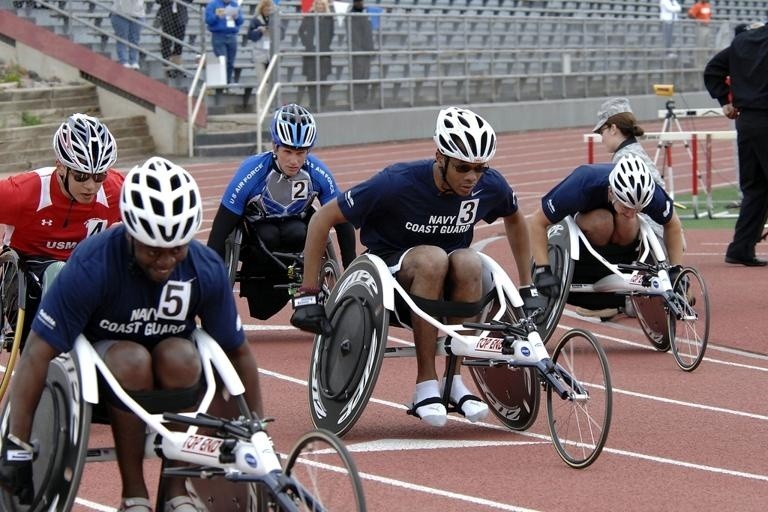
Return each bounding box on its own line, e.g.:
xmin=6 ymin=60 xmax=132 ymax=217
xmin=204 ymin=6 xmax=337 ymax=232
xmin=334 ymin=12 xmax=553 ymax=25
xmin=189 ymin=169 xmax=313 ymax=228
xmin=0 ymin=0 xmax=768 ymax=114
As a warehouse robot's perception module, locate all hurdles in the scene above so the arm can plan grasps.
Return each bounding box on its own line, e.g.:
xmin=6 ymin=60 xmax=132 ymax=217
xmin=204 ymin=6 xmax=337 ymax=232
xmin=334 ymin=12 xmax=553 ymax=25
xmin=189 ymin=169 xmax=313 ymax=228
xmin=582 ymin=109 xmax=743 ymax=219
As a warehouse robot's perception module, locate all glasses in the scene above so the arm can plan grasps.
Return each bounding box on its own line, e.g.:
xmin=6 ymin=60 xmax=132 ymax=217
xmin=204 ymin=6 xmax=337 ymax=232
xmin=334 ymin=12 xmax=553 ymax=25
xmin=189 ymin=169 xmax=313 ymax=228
xmin=74 ymin=173 xmax=107 ymax=182
xmin=455 ymin=164 xmax=489 ymax=172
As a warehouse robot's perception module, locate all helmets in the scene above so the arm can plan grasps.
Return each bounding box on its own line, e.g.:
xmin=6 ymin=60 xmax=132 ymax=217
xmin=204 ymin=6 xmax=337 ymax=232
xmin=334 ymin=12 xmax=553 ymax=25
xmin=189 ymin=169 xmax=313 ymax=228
xmin=53 ymin=113 xmax=117 ymax=174
xmin=119 ymin=156 xmax=203 ymax=248
xmin=271 ymin=103 xmax=317 ymax=150
xmin=609 ymin=153 xmax=655 ymax=210
xmin=433 ymin=107 xmax=497 ymax=163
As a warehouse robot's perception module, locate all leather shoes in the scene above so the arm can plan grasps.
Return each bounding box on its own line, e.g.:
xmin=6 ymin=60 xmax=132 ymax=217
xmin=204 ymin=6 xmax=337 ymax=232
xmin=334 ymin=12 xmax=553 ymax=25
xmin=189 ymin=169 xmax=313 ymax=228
xmin=725 ymin=251 xmax=767 ymax=266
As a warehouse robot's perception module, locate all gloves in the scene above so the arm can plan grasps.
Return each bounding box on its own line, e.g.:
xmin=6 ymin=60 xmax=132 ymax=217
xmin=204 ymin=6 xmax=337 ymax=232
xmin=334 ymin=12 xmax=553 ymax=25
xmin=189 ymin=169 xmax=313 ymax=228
xmin=519 ymin=264 xmax=560 ymax=324
xmin=0 ymin=433 xmax=34 ymax=504
xmin=290 ymin=292 xmax=333 ymax=336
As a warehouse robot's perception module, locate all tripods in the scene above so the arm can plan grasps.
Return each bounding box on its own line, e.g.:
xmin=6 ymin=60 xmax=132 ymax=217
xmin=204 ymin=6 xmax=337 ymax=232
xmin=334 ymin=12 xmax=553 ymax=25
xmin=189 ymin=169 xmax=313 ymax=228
xmin=653 ymin=100 xmax=714 ymax=217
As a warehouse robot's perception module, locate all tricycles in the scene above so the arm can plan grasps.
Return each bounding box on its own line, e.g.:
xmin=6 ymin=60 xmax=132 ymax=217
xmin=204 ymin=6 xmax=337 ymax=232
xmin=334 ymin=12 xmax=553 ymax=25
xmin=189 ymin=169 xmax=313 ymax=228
xmin=309 ymin=252 xmax=613 ymax=469
xmin=0 ymin=327 xmax=368 ymax=512
xmin=0 ymin=247 xmax=58 ymax=404
xmin=526 ymin=213 xmax=710 ymax=372
xmin=224 ymin=205 xmax=340 ymax=315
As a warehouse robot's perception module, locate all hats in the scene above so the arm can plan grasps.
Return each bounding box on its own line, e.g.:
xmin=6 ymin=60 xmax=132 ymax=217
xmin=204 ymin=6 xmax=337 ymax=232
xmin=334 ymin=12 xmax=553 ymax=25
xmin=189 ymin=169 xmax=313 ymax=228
xmin=592 ymin=98 xmax=631 ymax=133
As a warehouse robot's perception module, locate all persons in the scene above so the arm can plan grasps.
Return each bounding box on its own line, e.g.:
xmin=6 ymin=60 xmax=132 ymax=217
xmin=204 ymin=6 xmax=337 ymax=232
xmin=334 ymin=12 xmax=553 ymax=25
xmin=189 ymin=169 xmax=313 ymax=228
xmin=106 ymin=0 xmax=376 ymax=112
xmin=593 ymin=96 xmax=695 ymax=318
xmin=726 ymin=24 xmax=749 ymax=105
xmin=688 ymin=0 xmax=712 ymax=67
xmin=0 ymin=156 xmax=264 ymax=512
xmin=206 ymin=103 xmax=357 ymax=272
xmin=528 ymin=156 xmax=689 ymax=317
xmin=290 ymin=107 xmax=544 ymax=426
xmin=704 ymin=22 xmax=768 ymax=266
xmin=0 ymin=113 xmax=124 ymax=357
xmin=660 ymin=0 xmax=682 ymax=58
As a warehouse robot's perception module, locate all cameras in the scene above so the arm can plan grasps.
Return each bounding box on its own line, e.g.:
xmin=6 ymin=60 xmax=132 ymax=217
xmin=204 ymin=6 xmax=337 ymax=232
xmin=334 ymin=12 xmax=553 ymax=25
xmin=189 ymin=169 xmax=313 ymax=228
xmin=652 ymin=85 xmax=675 ymax=98
